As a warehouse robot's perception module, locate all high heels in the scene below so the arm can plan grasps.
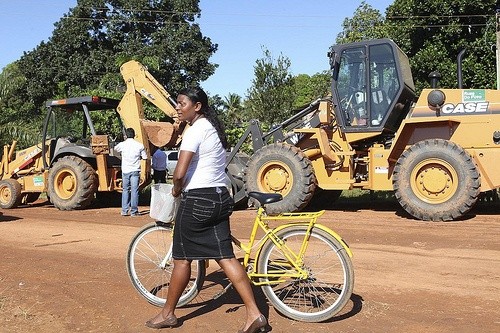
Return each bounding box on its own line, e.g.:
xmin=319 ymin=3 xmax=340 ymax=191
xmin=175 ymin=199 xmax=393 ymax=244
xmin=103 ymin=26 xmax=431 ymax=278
xmin=237 ymin=314 xmax=268 ymax=333
xmin=144 ymin=315 xmax=177 ymax=328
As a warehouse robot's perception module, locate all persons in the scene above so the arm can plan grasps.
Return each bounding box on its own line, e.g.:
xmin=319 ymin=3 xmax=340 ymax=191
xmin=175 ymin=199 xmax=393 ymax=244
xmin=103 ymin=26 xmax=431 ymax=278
xmin=114 ymin=128 xmax=148 ymax=217
xmin=144 ymin=86 xmax=267 ymax=333
xmin=152 ymin=146 xmax=167 ymax=184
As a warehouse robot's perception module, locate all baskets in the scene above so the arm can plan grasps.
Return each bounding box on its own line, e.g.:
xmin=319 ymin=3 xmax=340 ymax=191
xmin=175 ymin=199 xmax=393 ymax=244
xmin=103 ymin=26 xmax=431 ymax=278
xmin=149 ymin=183 xmax=181 ymax=223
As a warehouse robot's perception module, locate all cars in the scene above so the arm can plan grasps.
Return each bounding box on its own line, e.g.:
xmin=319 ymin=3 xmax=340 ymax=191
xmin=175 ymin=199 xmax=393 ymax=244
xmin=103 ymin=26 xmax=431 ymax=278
xmin=226 ymin=152 xmax=250 ymax=179
xmin=164 ymin=150 xmax=180 ymax=180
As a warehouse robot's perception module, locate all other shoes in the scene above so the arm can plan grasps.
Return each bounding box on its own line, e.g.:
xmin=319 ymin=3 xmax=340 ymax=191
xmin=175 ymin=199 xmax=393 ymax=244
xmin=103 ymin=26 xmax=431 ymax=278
xmin=131 ymin=214 xmax=135 ymax=216
xmin=121 ymin=213 xmax=128 ymax=215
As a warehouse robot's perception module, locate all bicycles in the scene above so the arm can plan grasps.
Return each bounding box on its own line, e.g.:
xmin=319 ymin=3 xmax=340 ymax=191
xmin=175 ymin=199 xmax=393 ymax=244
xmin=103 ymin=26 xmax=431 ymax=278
xmin=125 ymin=183 xmax=354 ymax=323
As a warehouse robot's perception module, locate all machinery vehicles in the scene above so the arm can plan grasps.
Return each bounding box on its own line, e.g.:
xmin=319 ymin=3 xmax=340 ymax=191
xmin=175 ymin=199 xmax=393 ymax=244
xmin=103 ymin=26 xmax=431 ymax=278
xmin=0 ymin=60 xmax=193 ymax=211
xmin=225 ymin=39 xmax=500 ymax=222
